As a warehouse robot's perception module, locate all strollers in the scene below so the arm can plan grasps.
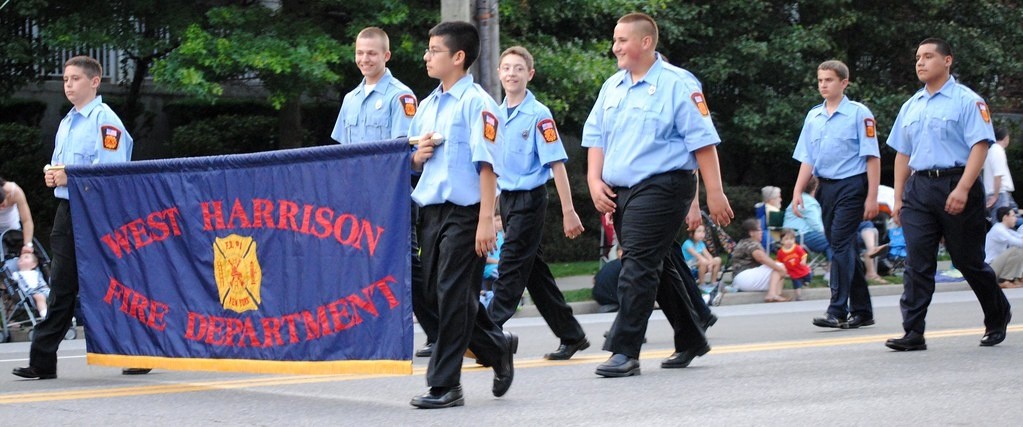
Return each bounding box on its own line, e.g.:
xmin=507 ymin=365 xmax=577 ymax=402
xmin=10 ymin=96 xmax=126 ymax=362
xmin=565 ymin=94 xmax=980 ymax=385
xmin=0 ymin=229 xmax=80 ymax=343
xmin=699 ymin=210 xmax=738 ymax=284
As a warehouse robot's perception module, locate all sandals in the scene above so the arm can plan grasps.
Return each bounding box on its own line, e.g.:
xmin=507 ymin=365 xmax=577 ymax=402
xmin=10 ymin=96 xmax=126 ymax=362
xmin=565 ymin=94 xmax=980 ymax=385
xmin=764 ymin=293 xmax=794 ymax=304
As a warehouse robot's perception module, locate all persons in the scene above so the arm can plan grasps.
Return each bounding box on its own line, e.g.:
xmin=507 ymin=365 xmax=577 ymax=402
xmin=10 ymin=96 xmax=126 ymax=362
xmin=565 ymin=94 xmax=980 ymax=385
xmin=883 ymin=37 xmax=1012 ymax=351
xmin=476 ymin=46 xmax=591 ymax=367
xmin=13 ymin=253 xmax=50 ymax=319
xmin=481 ymin=127 xmax=1023 ymax=335
xmin=330 ymin=26 xmax=417 ymax=141
xmin=580 ymin=12 xmax=734 ymax=377
xmin=407 ymin=20 xmax=520 ymax=410
xmin=12 ymin=55 xmax=152 ymax=379
xmin=791 ymin=59 xmax=880 ymax=329
xmin=0 ymin=177 xmax=34 ymax=255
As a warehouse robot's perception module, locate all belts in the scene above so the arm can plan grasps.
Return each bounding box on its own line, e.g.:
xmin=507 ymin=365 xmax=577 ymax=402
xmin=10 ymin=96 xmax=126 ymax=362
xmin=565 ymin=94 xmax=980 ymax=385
xmin=656 ymin=170 xmax=694 ymax=176
xmin=915 ymin=167 xmax=964 ymax=178
xmin=818 ymin=172 xmax=867 ymax=184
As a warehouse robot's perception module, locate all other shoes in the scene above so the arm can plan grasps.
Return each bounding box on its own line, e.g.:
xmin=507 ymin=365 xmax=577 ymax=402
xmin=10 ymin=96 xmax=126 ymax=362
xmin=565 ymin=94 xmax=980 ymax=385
xmin=794 ymin=294 xmax=805 ymax=301
xmin=699 ymin=282 xmax=717 ymax=293
xmin=823 ymin=272 xmax=831 ymax=286
xmin=999 ymin=279 xmax=1023 ymax=288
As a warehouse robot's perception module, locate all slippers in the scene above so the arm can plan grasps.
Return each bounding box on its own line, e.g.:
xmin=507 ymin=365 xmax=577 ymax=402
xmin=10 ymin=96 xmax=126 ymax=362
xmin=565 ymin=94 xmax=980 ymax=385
xmin=865 ymin=276 xmax=887 ymax=285
xmin=869 ymin=244 xmax=890 ymax=258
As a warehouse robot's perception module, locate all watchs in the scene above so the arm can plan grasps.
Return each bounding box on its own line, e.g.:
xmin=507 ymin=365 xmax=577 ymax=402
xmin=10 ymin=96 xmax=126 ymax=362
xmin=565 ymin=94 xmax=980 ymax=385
xmin=25 ymin=242 xmax=33 ymax=248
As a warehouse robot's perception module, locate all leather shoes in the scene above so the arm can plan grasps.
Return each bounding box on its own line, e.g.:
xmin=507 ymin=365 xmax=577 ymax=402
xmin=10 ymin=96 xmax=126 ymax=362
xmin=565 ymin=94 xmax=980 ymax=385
xmin=12 ymin=366 xmax=58 ymax=380
xmin=492 ymin=331 xmax=518 ymax=396
xmin=848 ymin=315 xmax=874 ymax=329
xmin=595 ymin=354 xmax=640 ymax=376
xmin=123 ymin=368 xmax=152 ymax=375
xmin=979 ymin=311 xmax=1012 ymax=345
xmin=544 ymin=336 xmax=590 ymax=358
xmin=416 ymin=344 xmax=434 ymax=357
xmin=411 ymin=385 xmax=465 ymax=408
xmin=813 ymin=312 xmax=849 ymax=329
xmin=604 ymin=331 xmax=646 ymax=344
xmin=698 ymin=314 xmax=718 ymax=333
xmin=886 ymin=330 xmax=926 ymax=351
xmin=661 ymin=345 xmax=710 ymax=368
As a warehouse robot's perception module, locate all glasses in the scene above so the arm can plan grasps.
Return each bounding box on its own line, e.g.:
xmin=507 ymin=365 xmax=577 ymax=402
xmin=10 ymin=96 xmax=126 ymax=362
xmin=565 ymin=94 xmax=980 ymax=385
xmin=752 ymin=230 xmax=764 ymax=233
